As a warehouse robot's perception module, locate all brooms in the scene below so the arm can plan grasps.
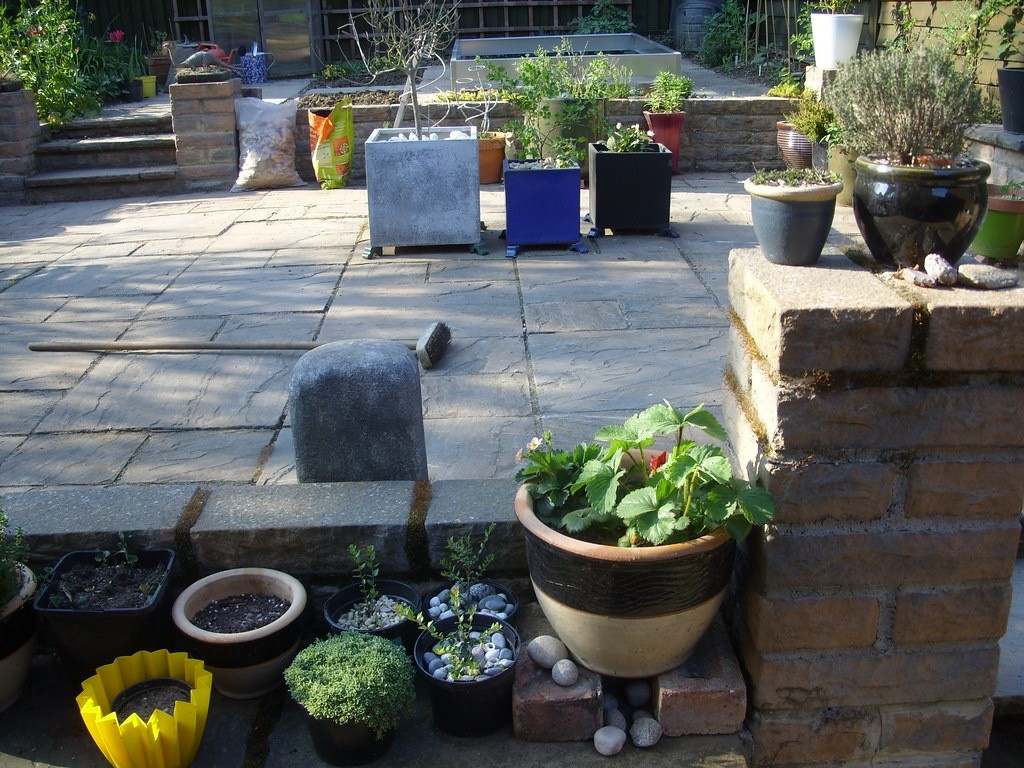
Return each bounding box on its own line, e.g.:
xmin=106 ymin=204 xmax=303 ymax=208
xmin=25 ymin=319 xmax=452 ymax=371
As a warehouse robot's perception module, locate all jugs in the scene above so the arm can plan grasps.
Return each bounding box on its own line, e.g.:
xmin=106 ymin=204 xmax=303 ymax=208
xmin=230 ymin=41 xmax=276 ymax=85
xmin=198 ymin=43 xmax=235 ymax=65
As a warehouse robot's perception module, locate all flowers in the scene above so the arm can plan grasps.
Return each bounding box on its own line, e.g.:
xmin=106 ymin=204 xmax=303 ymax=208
xmin=514 ymin=391 xmax=783 ymax=548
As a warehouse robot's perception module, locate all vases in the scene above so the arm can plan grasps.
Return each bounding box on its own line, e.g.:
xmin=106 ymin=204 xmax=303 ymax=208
xmin=75 ymin=649 xmax=214 ymax=768
xmin=506 ymin=448 xmax=776 ymax=680
xmin=173 ymin=566 xmax=309 ymax=701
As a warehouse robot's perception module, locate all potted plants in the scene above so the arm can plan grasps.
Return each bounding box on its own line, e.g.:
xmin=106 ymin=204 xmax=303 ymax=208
xmin=355 ymin=0 xmax=695 ymax=258
xmin=741 ymin=0 xmax=1024 ymax=269
xmin=283 ymin=518 xmax=522 ymax=768
xmin=0 ymin=502 xmax=176 ymax=716
xmin=121 ymin=30 xmax=171 ymax=104
